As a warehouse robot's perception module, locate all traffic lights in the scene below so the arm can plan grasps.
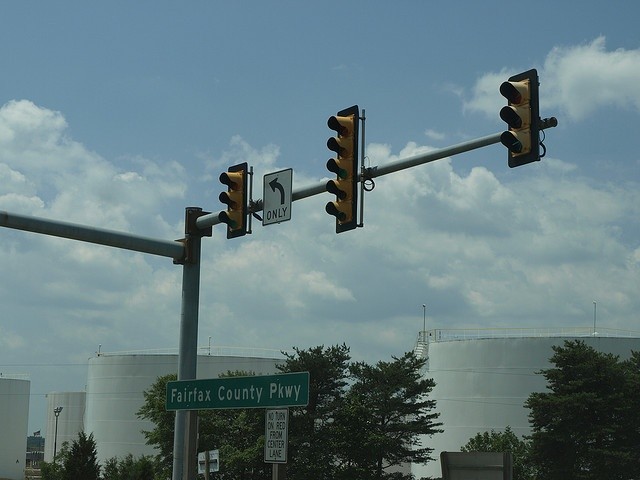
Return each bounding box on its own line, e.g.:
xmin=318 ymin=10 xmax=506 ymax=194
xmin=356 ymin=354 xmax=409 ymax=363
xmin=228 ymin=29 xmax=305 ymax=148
xmin=326 ymin=105 xmax=359 ymax=233
xmin=500 ymin=69 xmax=539 ymax=168
xmin=219 ymin=163 xmax=247 ymax=239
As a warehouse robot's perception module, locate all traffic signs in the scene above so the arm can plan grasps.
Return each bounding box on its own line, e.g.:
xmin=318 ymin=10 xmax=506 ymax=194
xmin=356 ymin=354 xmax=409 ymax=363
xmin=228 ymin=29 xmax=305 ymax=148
xmin=166 ymin=372 xmax=309 ymax=411
xmin=262 ymin=167 xmax=292 ymax=226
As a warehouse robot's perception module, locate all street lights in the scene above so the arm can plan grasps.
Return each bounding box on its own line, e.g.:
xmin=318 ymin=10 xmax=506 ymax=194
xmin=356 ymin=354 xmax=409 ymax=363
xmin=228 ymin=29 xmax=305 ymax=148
xmin=53 ymin=406 xmax=63 ymax=464
